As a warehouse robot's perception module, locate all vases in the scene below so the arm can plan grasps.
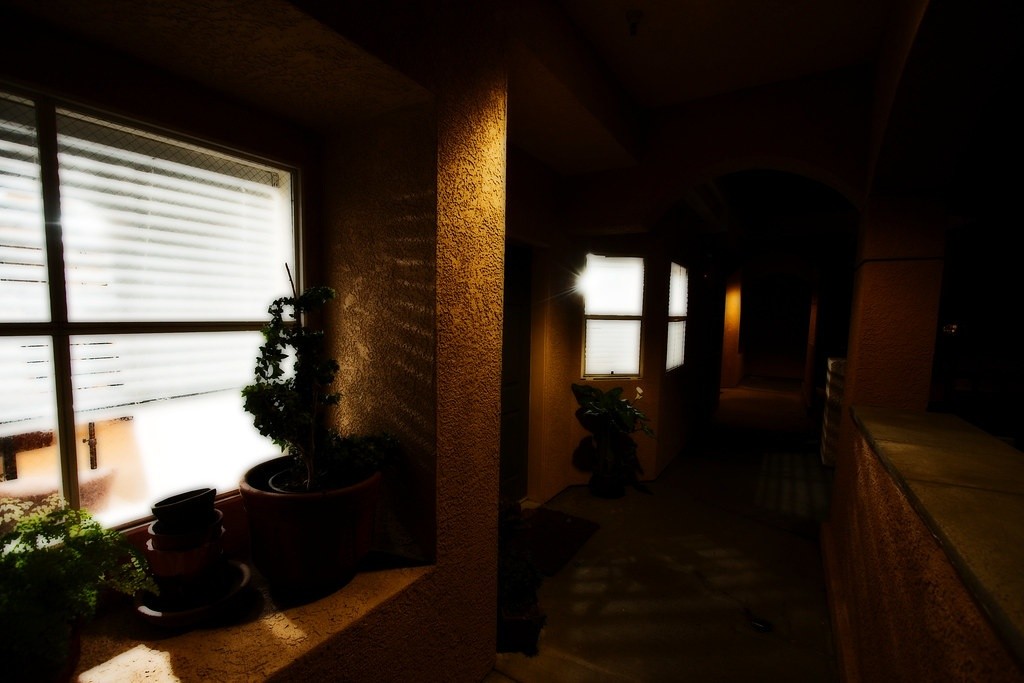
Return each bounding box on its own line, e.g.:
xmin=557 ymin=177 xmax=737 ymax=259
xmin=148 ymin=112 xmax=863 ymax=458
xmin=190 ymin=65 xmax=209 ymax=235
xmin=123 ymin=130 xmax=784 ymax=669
xmin=145 ymin=489 xmax=227 ymax=588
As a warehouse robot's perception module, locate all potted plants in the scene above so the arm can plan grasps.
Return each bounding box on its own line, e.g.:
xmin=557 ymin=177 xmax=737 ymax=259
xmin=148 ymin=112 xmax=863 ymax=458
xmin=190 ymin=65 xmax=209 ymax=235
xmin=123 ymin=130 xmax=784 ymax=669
xmin=0 ymin=505 xmax=160 ymax=683
xmin=497 ymin=546 xmax=546 ymax=656
xmin=239 ymin=281 xmax=400 ymax=579
xmin=571 ymin=382 xmax=656 ymax=500
